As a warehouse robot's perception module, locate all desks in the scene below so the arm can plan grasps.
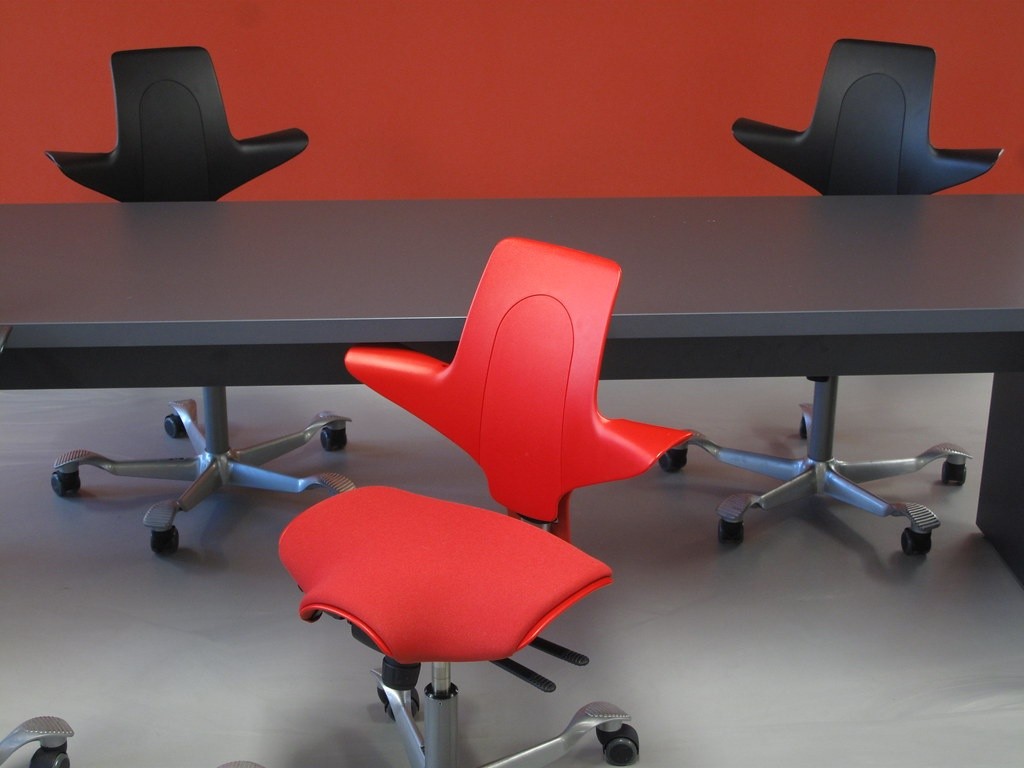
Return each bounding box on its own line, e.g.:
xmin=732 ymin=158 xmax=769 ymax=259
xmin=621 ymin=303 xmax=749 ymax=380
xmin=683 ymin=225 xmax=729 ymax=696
xmin=0 ymin=194 xmax=1024 ymax=581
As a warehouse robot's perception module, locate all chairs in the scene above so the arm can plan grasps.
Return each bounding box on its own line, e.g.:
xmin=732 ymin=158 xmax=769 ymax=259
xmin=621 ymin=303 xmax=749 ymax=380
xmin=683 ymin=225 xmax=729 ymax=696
xmin=46 ymin=36 xmax=1005 ymax=768
